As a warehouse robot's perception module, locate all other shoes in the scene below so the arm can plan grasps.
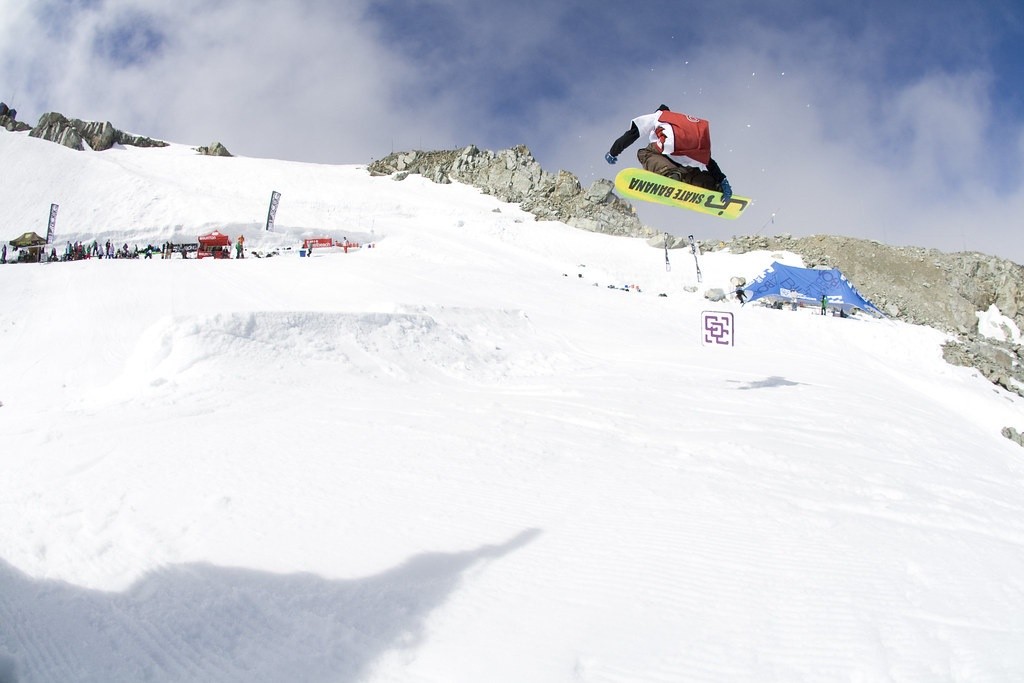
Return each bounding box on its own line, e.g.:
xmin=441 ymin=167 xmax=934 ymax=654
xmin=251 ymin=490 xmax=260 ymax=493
xmin=668 ymin=173 xmax=679 ymax=181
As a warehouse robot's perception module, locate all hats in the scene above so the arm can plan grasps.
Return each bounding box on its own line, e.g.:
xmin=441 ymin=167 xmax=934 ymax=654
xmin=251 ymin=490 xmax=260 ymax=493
xmin=654 ymin=104 xmax=669 ymax=112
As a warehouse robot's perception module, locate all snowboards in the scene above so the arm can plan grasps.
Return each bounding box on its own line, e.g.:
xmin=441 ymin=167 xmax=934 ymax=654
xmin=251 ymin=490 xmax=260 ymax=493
xmin=614 ymin=167 xmax=752 ymax=221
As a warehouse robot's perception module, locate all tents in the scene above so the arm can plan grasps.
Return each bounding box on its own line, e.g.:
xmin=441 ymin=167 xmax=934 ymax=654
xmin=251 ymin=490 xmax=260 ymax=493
xmin=729 ymin=261 xmax=887 ymax=320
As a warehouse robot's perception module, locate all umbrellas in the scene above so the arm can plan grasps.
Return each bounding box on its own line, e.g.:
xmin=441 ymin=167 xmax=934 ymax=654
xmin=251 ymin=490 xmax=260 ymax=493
xmin=9 ymin=232 xmax=46 ymax=251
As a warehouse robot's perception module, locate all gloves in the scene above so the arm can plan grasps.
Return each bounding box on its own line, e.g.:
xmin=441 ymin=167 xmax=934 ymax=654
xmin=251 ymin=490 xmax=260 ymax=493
xmin=720 ymin=178 xmax=732 ymax=203
xmin=605 ymin=152 xmax=617 ymax=164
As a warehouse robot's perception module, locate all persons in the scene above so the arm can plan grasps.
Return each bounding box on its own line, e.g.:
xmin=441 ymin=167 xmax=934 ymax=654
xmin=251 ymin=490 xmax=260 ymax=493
xmin=821 ymin=294 xmax=828 ymax=315
xmin=182 ymin=248 xmax=187 ymax=258
xmin=38 ymin=239 xmax=140 ymax=263
xmin=236 ymin=235 xmax=244 ymax=259
xmin=1 ymin=244 xmax=7 ymax=262
xmin=307 ymin=243 xmax=312 ymax=257
xmin=160 ymin=241 xmax=174 ymax=259
xmin=144 ymin=244 xmax=154 ymax=259
xmin=223 ymin=246 xmax=228 ymax=252
xmin=735 ymin=282 xmax=747 ymax=304
xmin=343 ymin=236 xmax=349 ymax=253
xmin=605 ymin=104 xmax=732 ymax=204
